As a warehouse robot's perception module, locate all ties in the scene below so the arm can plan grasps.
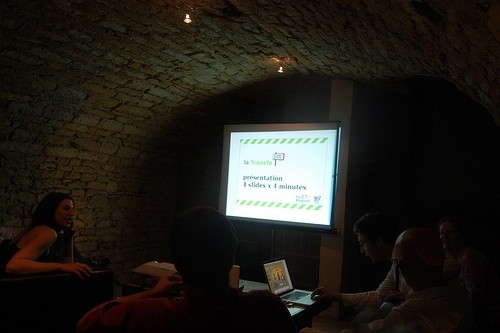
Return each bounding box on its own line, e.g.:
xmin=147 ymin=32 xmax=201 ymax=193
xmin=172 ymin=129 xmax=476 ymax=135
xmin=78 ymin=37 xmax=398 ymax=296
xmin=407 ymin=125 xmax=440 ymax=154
xmin=395 ymin=262 xmax=400 ymax=291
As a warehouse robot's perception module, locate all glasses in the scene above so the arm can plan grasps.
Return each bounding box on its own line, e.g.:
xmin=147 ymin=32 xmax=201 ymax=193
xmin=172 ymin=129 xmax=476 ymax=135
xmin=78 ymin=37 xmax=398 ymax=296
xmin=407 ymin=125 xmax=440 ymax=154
xmin=359 ymin=238 xmax=371 ymax=248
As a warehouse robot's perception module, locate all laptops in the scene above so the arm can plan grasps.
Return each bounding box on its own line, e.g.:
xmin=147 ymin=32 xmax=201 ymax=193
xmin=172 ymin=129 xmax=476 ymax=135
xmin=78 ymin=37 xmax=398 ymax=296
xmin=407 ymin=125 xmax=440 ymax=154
xmin=261 ymin=257 xmax=319 ymax=307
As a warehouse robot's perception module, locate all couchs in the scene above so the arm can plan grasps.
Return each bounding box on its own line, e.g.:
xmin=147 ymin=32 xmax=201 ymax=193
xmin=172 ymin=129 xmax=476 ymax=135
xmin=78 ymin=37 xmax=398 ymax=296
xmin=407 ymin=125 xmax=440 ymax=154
xmin=0 ymin=267 xmax=115 ymax=333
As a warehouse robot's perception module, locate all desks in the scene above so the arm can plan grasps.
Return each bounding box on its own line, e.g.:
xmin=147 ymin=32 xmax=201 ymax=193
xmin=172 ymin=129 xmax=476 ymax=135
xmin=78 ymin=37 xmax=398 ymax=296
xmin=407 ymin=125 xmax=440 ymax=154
xmin=237 ymin=278 xmax=332 ymax=333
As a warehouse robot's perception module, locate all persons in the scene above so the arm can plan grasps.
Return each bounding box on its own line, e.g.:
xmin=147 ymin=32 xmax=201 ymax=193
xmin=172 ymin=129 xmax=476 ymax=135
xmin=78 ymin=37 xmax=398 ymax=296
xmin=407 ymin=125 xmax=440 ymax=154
xmin=76 ymin=205 xmax=292 ymax=333
xmin=0 ymin=192 xmax=94 ymax=280
xmin=310 ymin=212 xmax=495 ymax=333
